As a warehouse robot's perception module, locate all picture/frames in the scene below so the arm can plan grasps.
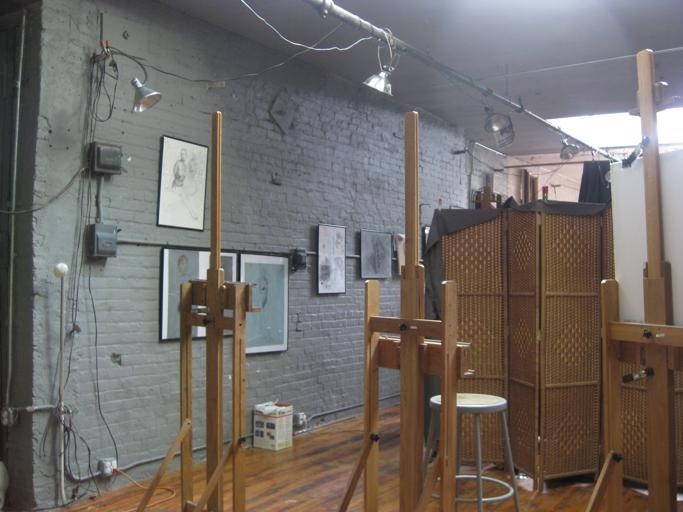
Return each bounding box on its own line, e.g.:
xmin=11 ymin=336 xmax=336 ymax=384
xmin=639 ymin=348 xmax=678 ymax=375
xmin=360 ymin=229 xmax=392 ymax=279
xmin=156 ymin=135 xmax=209 ymax=231
xmin=240 ymin=251 xmax=289 ymax=354
xmin=160 ymin=247 xmax=238 ymax=341
xmin=317 ymin=223 xmax=346 ymax=295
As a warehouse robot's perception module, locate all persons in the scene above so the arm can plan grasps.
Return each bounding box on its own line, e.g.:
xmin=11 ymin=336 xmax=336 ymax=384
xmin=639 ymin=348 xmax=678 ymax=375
xmin=172 ymin=148 xmax=199 ymax=220
xmin=174 ymin=256 xmax=192 ymax=284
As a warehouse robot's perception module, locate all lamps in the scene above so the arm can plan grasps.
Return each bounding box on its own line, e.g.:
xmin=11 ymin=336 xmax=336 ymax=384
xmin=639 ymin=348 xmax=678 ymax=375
xmin=360 ymin=64 xmax=401 ymax=97
xmin=559 ymin=137 xmax=580 ymax=160
xmin=484 ymin=105 xmax=511 ymax=133
xmin=130 ymin=76 xmax=163 ymax=113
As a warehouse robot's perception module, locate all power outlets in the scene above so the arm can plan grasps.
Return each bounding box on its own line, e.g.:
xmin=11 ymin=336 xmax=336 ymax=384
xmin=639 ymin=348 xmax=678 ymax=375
xmin=100 ymin=457 xmax=118 ymax=478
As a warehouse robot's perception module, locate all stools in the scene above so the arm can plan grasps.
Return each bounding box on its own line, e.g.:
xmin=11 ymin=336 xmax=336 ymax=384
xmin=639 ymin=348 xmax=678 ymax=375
xmin=421 ymin=393 xmax=521 ymax=512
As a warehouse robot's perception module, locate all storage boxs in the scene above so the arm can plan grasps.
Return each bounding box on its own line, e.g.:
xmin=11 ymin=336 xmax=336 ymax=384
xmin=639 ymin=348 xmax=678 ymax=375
xmin=253 ymin=405 xmax=293 ymax=451
